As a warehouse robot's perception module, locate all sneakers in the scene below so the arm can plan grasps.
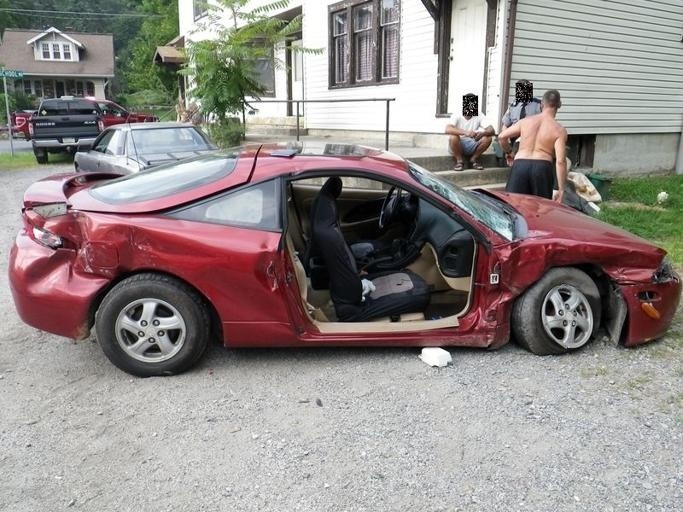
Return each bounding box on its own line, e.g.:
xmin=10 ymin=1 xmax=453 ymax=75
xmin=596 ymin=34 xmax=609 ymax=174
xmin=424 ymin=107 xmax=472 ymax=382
xmin=453 ymin=162 xmax=464 ymax=171
xmin=470 ymin=159 xmax=483 ymax=170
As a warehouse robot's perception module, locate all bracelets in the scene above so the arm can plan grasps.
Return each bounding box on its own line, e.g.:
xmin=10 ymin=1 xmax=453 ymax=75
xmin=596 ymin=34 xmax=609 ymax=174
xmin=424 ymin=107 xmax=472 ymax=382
xmin=505 ymin=149 xmax=512 ymax=154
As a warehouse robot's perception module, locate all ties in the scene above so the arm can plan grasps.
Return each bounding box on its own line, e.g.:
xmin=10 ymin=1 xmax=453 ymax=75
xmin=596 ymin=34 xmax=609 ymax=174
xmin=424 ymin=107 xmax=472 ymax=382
xmin=519 ymin=103 xmax=527 ymax=120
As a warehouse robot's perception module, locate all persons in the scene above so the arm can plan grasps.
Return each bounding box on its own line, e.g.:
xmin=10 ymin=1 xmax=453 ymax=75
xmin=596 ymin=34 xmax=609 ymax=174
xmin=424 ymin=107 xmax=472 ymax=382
xmin=445 ymin=93 xmax=495 ymax=170
xmin=498 ymin=90 xmax=567 ymax=204
xmin=178 ymin=97 xmax=205 ymax=141
xmin=501 ymin=79 xmax=541 ymax=167
xmin=174 ymin=104 xmax=189 ymax=139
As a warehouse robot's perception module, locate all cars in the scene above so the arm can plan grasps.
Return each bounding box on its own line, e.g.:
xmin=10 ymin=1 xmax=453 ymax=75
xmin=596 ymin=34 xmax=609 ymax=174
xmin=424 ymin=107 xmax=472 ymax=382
xmin=74 ymin=121 xmax=222 ymax=176
xmin=28 ymin=97 xmax=104 ymax=163
xmin=6 ymin=140 xmax=683 ymax=378
xmin=12 ymin=96 xmax=161 ymax=141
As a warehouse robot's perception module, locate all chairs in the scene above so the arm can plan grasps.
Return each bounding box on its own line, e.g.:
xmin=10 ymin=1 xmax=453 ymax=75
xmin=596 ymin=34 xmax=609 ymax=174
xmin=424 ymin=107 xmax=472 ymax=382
xmin=284 ymin=171 xmax=432 ymax=321
